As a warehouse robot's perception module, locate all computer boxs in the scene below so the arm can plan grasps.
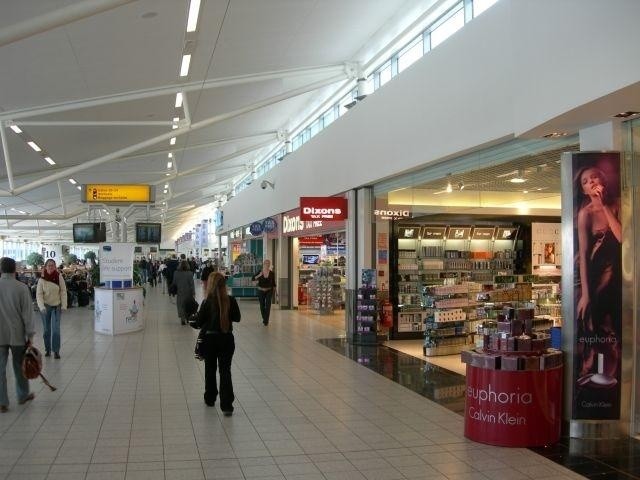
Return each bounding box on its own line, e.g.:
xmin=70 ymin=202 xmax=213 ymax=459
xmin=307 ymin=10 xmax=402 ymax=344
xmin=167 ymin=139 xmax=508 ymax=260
xmin=261 ymin=182 xmax=267 ymax=190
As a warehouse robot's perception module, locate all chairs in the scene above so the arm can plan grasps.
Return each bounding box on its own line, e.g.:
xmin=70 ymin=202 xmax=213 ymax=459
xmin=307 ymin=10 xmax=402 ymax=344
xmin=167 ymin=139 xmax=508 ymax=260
xmin=222 ymin=410 xmax=233 ymax=417
xmin=181 ymin=321 xmax=190 ymax=326
xmin=0 ymin=404 xmax=9 ymax=413
xmin=204 ymin=399 xmax=216 ymax=407
xmin=262 ymin=320 xmax=269 ymax=327
xmin=17 ymin=393 xmax=35 ymax=405
xmin=44 ymin=350 xmax=61 ymax=361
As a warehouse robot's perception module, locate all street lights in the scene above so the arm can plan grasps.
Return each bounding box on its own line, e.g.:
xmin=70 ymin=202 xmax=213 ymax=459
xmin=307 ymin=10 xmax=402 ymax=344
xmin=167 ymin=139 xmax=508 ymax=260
xmin=22 ymin=345 xmax=44 ymax=381
xmin=193 ymin=300 xmax=208 ymax=362
xmin=189 ymin=298 xmax=199 ymax=316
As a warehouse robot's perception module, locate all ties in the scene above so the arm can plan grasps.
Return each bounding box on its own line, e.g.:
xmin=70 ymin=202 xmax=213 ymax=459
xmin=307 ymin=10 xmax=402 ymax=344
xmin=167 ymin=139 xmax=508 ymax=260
xmin=136 ymin=223 xmax=162 ymax=244
xmin=73 ymin=222 xmax=106 ymax=243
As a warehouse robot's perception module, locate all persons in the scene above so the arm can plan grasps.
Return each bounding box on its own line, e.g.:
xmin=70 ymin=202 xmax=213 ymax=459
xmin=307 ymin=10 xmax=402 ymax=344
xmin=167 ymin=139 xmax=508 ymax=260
xmin=252 ymin=259 xmax=276 ymax=325
xmin=573 ymin=166 xmax=623 ymax=377
xmin=0 ymin=254 xmax=214 ymax=413
xmin=545 ymin=244 xmax=554 ymax=263
xmin=189 ymin=272 xmax=241 ymax=416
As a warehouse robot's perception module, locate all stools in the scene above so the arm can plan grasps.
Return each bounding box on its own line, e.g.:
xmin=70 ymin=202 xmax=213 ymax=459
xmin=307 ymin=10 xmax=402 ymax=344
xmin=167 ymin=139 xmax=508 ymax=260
xmin=206 ymin=329 xmax=232 ymax=336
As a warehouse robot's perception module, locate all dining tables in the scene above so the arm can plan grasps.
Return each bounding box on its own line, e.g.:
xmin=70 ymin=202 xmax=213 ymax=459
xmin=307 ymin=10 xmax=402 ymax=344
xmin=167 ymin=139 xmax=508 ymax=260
xmin=25 ymin=251 xmax=44 ymax=271
